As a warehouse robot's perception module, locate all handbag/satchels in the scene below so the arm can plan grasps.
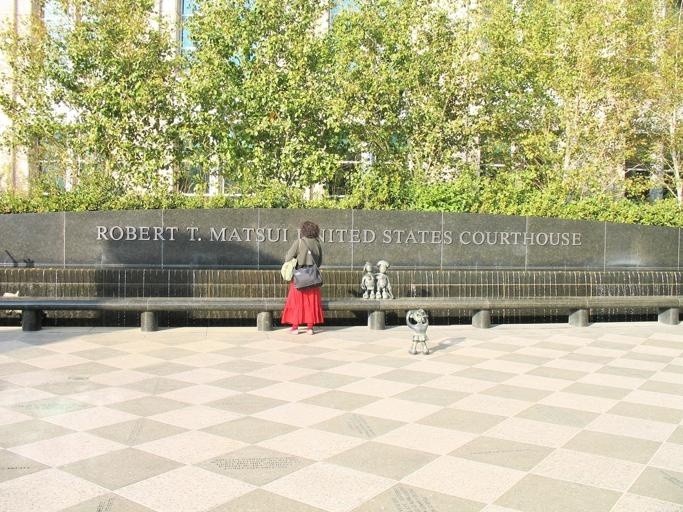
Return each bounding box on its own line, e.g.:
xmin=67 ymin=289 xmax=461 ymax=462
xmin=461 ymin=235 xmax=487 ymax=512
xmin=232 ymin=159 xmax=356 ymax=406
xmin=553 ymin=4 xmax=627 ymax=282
xmin=281 ymin=258 xmax=297 ymax=281
xmin=292 ymin=264 xmax=323 ymax=290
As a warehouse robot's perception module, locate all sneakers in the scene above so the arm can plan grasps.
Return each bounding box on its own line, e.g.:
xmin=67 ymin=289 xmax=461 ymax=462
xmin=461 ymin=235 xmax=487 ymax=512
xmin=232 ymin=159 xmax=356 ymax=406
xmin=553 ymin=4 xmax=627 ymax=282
xmin=305 ymin=329 xmax=314 ymax=335
xmin=288 ymin=330 xmax=299 ymax=335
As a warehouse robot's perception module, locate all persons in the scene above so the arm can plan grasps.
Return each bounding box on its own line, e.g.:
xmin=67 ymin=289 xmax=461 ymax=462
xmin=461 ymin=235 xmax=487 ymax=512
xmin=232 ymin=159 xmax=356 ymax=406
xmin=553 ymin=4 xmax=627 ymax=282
xmin=280 ymin=221 xmax=324 ymax=335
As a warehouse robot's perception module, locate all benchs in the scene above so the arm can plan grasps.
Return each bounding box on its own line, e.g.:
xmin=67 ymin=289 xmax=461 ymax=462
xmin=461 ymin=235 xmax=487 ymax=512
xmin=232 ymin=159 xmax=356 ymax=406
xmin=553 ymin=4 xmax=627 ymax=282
xmin=0 ymin=289 xmax=681 ymax=334
